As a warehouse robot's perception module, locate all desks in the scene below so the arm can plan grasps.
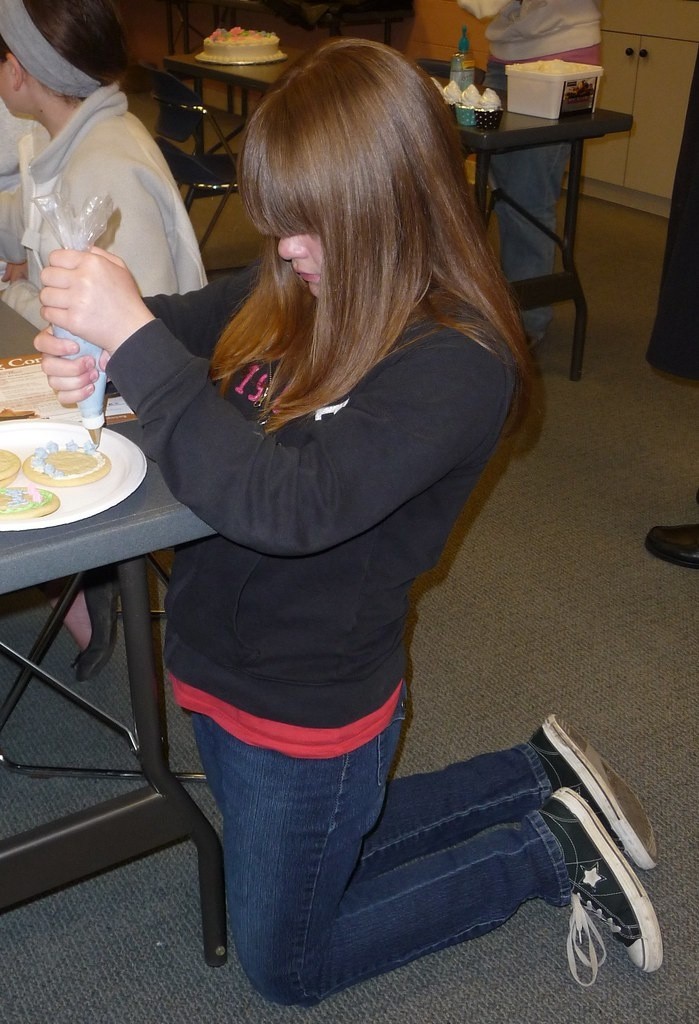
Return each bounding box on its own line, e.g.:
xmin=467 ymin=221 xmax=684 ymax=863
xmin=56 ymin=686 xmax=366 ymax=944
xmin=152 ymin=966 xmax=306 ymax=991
xmin=164 ymin=0 xmax=416 ymax=118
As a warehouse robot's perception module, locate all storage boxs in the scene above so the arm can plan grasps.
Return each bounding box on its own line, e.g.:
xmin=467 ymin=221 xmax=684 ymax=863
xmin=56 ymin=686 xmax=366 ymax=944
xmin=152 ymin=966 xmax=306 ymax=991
xmin=505 ymin=59 xmax=604 ymax=119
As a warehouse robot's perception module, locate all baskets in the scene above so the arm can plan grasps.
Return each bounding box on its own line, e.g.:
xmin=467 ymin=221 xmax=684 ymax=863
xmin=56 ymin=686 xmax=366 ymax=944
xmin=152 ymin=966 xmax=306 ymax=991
xmin=451 ymin=104 xmax=504 ymax=130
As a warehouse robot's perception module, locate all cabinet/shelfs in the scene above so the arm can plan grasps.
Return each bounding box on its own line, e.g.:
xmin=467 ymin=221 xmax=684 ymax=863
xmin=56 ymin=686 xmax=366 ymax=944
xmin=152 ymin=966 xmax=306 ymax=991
xmin=562 ymin=29 xmax=699 ymax=199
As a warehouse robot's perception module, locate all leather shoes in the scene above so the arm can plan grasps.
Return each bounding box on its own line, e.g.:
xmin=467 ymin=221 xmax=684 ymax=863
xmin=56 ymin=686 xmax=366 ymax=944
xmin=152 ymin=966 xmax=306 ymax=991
xmin=644 ymin=524 xmax=699 ymax=568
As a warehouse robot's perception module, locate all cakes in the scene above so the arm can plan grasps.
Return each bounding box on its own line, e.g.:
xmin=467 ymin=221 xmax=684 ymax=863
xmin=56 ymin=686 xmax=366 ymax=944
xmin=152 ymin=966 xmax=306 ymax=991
xmin=199 ymin=26 xmax=282 ymax=62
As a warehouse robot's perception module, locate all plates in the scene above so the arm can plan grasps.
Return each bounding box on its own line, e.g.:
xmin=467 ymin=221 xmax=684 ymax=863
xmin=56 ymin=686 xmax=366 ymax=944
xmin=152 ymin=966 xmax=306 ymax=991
xmin=0 ymin=420 xmax=147 ymax=530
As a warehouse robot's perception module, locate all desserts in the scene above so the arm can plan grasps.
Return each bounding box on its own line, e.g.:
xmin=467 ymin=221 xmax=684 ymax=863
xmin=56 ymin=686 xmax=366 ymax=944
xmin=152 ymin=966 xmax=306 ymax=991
xmin=431 ymin=77 xmax=504 ymax=130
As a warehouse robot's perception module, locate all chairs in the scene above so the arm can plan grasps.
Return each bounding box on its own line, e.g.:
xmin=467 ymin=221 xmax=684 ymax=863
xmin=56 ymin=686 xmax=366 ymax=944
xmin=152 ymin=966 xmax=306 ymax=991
xmin=139 ymin=61 xmax=242 ymax=252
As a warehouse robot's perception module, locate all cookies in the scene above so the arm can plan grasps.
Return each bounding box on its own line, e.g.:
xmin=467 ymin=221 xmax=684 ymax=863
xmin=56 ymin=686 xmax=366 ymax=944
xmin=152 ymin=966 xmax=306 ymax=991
xmin=21 ymin=450 xmax=111 ymax=487
xmin=0 ymin=486 xmax=61 ymax=519
xmin=0 ymin=448 xmax=21 ymax=488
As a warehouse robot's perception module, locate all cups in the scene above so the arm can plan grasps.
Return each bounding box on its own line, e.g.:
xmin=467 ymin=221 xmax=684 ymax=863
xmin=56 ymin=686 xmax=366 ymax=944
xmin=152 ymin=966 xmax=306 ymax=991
xmin=456 ymin=102 xmax=477 ymax=126
xmin=446 ymin=101 xmax=456 ymax=121
xmin=474 ymin=108 xmax=504 ymax=130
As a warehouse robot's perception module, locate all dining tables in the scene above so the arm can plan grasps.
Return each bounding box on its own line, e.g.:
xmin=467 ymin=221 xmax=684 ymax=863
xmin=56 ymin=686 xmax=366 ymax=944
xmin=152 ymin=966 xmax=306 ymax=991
xmin=0 ymin=300 xmax=228 ymax=968
xmin=163 ymin=53 xmax=633 ymax=383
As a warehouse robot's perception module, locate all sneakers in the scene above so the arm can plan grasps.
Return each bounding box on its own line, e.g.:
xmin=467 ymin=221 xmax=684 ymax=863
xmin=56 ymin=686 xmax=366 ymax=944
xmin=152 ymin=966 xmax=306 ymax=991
xmin=536 ymin=787 xmax=662 ymax=986
xmin=524 ymin=715 xmax=657 ymax=869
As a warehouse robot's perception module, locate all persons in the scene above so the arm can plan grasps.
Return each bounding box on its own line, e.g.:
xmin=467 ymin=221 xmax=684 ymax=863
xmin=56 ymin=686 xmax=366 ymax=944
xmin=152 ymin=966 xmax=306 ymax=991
xmin=453 ymin=0 xmax=603 ymax=348
xmin=31 ymin=38 xmax=664 ymax=1006
xmin=0 ymin=1 xmax=209 ymax=681
xmin=638 ymin=45 xmax=699 ymax=566
